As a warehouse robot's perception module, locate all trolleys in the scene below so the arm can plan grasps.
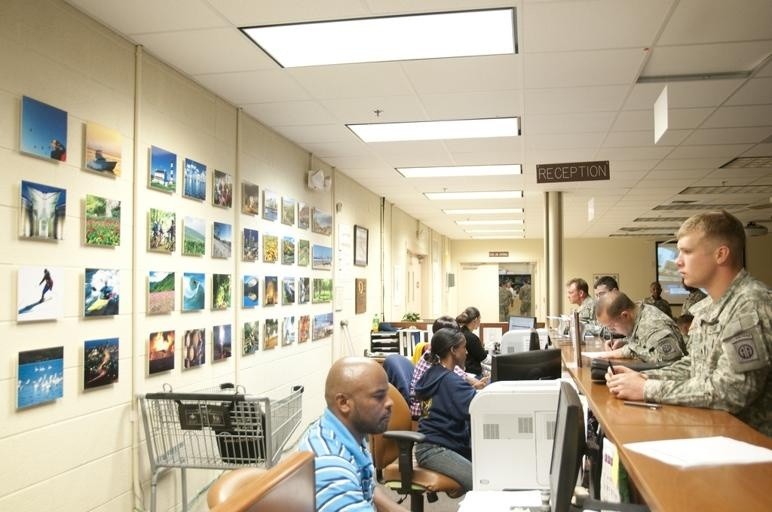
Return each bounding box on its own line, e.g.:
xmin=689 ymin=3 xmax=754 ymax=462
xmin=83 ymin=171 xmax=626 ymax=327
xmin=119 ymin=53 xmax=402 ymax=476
xmin=136 ymin=381 xmax=305 ymax=512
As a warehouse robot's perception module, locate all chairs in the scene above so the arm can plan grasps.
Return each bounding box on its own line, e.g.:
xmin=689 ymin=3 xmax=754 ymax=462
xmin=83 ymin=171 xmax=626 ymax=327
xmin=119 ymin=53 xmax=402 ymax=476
xmin=365 ymin=383 xmax=465 ymax=512
xmin=208 ymin=451 xmax=318 ymax=512
xmin=382 ymin=354 xmax=415 ymax=405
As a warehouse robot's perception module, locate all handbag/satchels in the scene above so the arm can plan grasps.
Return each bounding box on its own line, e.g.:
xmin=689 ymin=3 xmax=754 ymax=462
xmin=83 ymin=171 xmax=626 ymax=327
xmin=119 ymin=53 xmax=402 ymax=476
xmin=215 ymin=403 xmax=267 ymax=464
xmin=591 ymin=358 xmax=669 ymax=385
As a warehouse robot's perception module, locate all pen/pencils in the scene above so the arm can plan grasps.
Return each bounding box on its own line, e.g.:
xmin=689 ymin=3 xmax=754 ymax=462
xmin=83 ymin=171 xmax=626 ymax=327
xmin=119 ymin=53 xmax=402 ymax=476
xmin=610 ymin=333 xmax=613 ymax=351
xmin=624 ymin=401 xmax=663 ymax=408
xmin=608 ymin=360 xmax=616 ymax=375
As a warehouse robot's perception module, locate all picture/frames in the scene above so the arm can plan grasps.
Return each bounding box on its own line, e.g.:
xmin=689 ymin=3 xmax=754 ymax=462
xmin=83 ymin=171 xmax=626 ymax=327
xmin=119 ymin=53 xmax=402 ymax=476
xmin=352 ymin=223 xmax=371 ymax=269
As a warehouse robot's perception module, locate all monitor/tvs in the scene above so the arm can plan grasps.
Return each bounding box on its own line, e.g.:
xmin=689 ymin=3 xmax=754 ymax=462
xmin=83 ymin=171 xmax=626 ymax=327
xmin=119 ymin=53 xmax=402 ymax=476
xmin=509 ymin=315 xmax=535 ymax=329
xmin=491 ymin=349 xmax=561 ymax=380
xmin=548 ymin=381 xmax=584 ymax=512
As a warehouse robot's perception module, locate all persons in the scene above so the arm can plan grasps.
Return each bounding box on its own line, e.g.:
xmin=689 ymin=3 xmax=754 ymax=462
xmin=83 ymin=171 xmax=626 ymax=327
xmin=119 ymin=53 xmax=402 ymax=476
xmin=499 ymin=278 xmax=531 ymax=322
xmin=563 ymin=278 xmax=708 ymax=363
xmin=409 ymin=317 xmax=489 ymax=421
xmin=414 ymin=327 xmax=477 ymax=490
xmin=456 ymin=307 xmax=492 ymax=385
xmin=604 ymin=211 xmax=772 ymax=438
xmin=40 ymin=270 xmax=53 ymax=302
xmin=50 ymin=139 xmax=66 ymax=161
xmin=298 ymin=355 xmax=410 ymax=512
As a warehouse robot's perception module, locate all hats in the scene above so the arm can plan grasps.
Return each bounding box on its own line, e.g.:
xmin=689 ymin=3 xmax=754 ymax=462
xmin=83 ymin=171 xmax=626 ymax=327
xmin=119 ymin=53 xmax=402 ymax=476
xmin=379 ymin=323 xmax=398 ymax=331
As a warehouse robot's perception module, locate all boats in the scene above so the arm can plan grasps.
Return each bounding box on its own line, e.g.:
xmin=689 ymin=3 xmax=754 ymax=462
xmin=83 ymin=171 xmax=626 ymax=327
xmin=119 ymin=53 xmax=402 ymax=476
xmin=86 ymin=293 xmax=116 ymax=311
xmin=87 ymin=160 xmax=117 ymax=171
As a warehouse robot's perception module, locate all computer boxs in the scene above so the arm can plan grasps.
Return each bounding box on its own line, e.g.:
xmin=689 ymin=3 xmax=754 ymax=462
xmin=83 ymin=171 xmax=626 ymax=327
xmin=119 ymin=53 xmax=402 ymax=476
xmin=469 ymin=381 xmax=560 ymax=491
xmin=502 ymin=329 xmax=539 ymax=354
